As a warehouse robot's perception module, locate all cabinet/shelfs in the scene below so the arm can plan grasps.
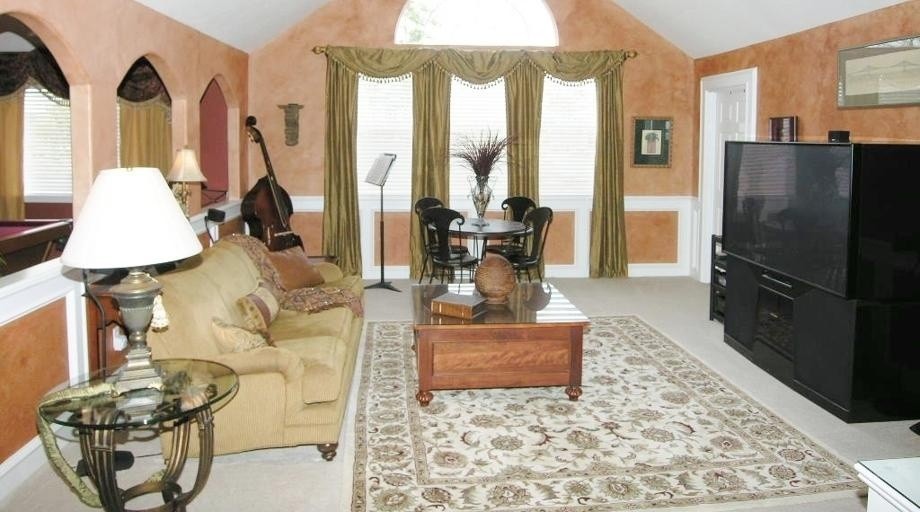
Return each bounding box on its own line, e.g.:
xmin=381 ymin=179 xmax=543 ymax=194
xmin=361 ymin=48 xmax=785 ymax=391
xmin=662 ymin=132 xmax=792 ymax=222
xmin=710 ymin=234 xmax=728 ymax=324
xmin=721 ymin=251 xmax=920 ymax=423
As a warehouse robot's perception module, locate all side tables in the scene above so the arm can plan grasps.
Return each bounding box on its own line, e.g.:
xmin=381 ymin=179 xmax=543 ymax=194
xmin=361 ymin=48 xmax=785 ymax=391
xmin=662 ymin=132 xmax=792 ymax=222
xmin=37 ymin=358 xmax=239 ymax=512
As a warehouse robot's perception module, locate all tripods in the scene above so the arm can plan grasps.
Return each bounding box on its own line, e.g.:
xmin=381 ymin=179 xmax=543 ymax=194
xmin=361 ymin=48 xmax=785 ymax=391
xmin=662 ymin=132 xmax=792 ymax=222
xmin=363 ymin=186 xmax=402 ymax=293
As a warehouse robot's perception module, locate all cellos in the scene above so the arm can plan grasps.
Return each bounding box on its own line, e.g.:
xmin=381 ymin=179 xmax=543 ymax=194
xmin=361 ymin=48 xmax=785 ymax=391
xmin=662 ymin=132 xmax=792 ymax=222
xmin=241 ymin=116 xmax=304 ymax=253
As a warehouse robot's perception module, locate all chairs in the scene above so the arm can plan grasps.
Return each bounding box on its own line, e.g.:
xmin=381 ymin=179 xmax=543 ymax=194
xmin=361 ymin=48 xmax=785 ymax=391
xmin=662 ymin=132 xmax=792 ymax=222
xmin=521 ymin=283 xmax=551 ymax=311
xmin=415 ymin=197 xmax=554 ymax=283
xmin=419 ymin=284 xmax=448 ymax=314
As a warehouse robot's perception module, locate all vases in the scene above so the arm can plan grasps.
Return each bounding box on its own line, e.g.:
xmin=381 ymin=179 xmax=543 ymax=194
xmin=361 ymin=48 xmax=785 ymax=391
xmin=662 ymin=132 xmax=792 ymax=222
xmin=471 ymin=176 xmax=492 ymax=227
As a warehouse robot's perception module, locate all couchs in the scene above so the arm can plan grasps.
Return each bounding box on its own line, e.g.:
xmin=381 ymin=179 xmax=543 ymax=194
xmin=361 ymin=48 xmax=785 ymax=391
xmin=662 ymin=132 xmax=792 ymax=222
xmin=146 ymin=233 xmax=365 ymax=467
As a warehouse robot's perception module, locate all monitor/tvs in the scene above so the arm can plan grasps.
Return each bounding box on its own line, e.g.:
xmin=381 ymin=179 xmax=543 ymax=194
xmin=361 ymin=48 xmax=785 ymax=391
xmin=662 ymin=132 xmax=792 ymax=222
xmin=722 ymin=141 xmax=860 ymax=299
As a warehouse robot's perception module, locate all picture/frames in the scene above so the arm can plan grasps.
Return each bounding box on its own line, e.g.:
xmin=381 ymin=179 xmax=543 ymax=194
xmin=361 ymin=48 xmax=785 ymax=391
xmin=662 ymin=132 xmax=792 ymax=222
xmin=630 ymin=116 xmax=673 ymax=169
xmin=836 ymin=36 xmax=920 ymax=108
xmin=769 ymin=116 xmax=797 ymax=142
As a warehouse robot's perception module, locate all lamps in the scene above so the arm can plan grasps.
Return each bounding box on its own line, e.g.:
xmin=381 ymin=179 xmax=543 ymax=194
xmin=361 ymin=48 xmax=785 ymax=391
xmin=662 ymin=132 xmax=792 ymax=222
xmin=60 ymin=167 xmax=204 ymax=399
xmin=392 ymin=0 xmax=560 ymax=48
xmin=165 ymin=145 xmax=208 ymax=222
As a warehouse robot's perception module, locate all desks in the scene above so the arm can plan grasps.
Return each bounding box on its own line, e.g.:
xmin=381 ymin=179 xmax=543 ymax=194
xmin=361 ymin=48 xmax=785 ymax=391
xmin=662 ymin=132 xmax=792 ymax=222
xmin=0 ymin=218 xmax=74 ymax=278
xmin=854 ymin=456 xmax=920 ymax=512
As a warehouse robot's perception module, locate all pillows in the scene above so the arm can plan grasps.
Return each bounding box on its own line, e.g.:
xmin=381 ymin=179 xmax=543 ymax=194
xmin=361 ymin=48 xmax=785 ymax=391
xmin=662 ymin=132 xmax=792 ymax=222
xmin=210 ymin=316 xmax=276 ymax=357
xmin=264 ymin=245 xmax=325 ymax=293
xmin=237 ymin=281 xmax=279 ymax=333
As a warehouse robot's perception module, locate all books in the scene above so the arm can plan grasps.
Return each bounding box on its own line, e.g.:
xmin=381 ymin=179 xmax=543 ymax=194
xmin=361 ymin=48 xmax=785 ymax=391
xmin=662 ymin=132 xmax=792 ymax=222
xmin=430 ymin=292 xmax=489 ymax=320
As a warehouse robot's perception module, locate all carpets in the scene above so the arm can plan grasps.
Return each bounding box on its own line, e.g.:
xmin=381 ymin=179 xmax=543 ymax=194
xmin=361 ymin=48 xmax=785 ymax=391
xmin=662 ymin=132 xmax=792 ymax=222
xmin=351 ymin=315 xmax=868 ymax=512
